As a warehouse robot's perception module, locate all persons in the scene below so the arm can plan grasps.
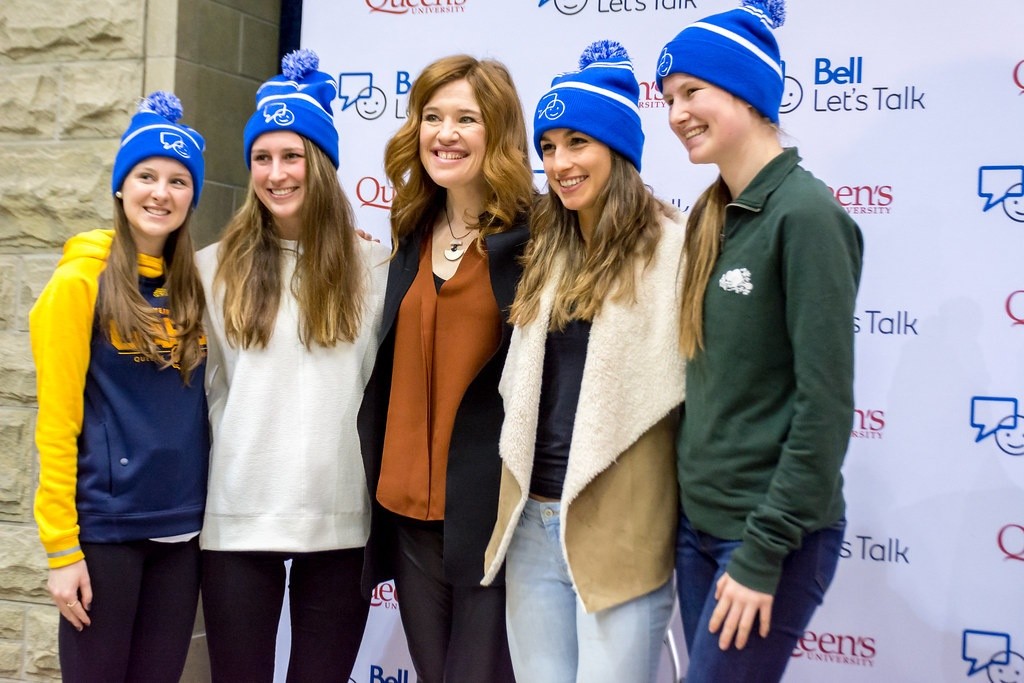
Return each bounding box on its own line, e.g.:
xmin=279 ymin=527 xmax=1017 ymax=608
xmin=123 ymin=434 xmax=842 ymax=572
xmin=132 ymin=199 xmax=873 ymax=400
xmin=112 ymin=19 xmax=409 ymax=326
xmin=28 ymin=92 xmax=380 ymax=683
xmin=654 ymin=0 xmax=863 ymax=682
xmin=356 ymin=57 xmax=542 ymax=683
xmin=192 ymin=47 xmax=392 ymax=683
xmin=482 ymin=42 xmax=682 ymax=683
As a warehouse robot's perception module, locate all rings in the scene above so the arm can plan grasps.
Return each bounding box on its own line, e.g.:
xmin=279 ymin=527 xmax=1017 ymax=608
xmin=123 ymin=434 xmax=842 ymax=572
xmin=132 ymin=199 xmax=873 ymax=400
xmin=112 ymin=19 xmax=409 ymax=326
xmin=67 ymin=600 xmax=77 ymax=608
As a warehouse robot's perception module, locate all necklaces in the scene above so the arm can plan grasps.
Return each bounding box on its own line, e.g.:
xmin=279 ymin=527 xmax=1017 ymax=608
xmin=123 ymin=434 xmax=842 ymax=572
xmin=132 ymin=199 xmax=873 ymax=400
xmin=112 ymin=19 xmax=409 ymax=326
xmin=445 ymin=209 xmax=479 ymax=261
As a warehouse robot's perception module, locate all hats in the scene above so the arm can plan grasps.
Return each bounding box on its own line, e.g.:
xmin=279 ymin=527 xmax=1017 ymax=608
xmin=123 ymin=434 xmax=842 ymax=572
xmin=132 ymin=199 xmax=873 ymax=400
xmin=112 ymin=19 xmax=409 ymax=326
xmin=655 ymin=0 xmax=787 ymax=125
xmin=534 ymin=40 xmax=645 ymax=173
xmin=112 ymin=90 xmax=205 ymax=210
xmin=242 ymin=49 xmax=340 ymax=170
